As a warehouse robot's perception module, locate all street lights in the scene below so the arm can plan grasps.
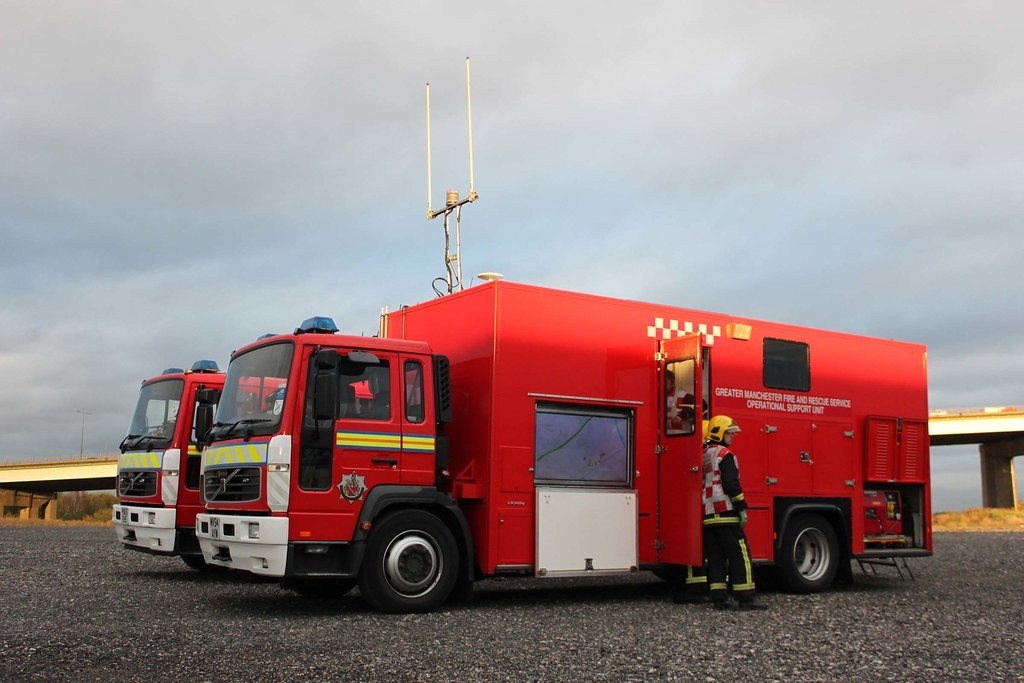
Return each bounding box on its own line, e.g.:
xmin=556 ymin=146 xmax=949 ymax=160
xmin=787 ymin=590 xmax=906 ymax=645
xmin=75 ymin=409 xmax=93 ymax=459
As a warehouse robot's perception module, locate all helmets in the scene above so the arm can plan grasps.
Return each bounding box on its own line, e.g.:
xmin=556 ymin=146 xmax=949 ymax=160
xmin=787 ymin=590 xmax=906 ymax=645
xmin=706 ymin=415 xmax=741 ymax=442
xmin=691 ymin=420 xmax=709 ymax=443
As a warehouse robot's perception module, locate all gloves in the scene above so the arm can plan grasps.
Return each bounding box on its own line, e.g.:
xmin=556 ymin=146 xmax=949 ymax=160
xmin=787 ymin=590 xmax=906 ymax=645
xmin=739 ymin=508 xmax=748 ymax=528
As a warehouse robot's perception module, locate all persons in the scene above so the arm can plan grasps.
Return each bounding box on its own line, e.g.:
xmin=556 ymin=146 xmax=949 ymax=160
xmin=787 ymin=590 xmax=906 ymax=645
xmin=665 ymin=369 xmax=768 ymax=611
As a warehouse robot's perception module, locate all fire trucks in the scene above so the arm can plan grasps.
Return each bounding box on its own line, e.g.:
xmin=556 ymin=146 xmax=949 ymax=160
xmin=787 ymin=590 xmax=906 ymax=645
xmin=112 ymin=360 xmax=376 ymax=576
xmin=194 ymin=271 xmax=933 ymax=610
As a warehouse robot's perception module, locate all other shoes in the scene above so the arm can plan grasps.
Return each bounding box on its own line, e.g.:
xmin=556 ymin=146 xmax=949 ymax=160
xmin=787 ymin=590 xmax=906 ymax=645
xmin=681 ymin=590 xmax=712 ymax=603
xmin=739 ymin=598 xmax=769 ymax=610
xmin=713 ymin=600 xmax=738 ymax=611
